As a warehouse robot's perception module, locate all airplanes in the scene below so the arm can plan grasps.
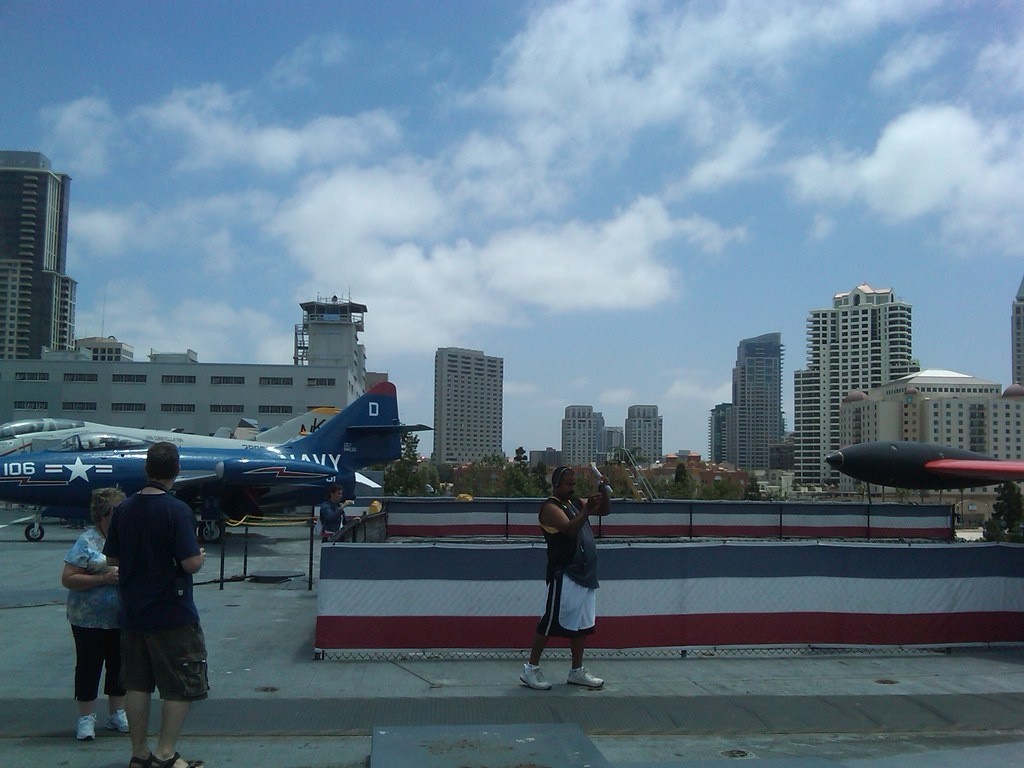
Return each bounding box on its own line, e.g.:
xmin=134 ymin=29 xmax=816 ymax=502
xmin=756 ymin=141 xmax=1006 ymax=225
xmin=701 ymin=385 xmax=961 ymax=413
xmin=823 ymin=439 xmax=1024 ymax=491
xmin=0 ymin=378 xmax=437 ymax=543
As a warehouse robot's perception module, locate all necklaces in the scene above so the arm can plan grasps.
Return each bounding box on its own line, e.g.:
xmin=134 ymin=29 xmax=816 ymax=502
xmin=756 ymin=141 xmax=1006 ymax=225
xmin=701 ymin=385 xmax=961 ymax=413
xmin=98 ymin=524 xmax=108 ymax=540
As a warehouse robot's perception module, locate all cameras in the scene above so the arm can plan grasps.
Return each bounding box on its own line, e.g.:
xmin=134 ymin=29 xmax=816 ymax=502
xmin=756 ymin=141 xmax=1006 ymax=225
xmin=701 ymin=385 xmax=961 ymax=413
xmin=351 ymin=501 xmax=354 ymax=504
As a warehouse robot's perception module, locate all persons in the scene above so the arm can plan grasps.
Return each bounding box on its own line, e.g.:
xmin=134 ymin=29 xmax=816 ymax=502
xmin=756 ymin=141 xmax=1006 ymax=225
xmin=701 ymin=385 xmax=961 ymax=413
xmin=319 ymin=485 xmax=361 ymax=542
xmin=520 ymin=466 xmax=612 ymax=690
xmin=62 ymin=488 xmax=130 ymax=740
xmin=102 ymin=442 xmax=210 ymax=768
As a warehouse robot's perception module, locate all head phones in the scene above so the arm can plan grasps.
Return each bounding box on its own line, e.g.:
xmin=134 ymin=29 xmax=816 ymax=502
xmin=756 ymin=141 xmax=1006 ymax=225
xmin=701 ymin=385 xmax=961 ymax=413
xmin=554 ymin=468 xmax=569 ymax=488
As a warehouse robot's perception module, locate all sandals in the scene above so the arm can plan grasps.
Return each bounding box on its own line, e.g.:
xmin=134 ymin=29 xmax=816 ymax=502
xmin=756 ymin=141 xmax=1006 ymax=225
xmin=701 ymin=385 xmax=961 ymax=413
xmin=151 ymin=752 xmax=204 ymax=768
xmin=129 ymin=755 xmax=153 ymax=768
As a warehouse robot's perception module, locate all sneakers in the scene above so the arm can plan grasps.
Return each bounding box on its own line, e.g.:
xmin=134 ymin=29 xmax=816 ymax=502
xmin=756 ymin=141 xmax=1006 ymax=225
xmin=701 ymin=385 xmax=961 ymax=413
xmin=520 ymin=663 xmax=553 ymax=690
xmin=105 ymin=710 xmax=131 ymax=733
xmin=567 ymin=666 xmax=604 ymax=687
xmin=77 ymin=715 xmax=97 ymax=741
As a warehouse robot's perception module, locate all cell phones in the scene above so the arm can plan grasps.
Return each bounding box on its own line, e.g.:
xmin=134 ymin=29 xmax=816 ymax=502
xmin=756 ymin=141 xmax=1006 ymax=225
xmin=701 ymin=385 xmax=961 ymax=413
xmin=598 ymin=483 xmax=603 ymax=492
